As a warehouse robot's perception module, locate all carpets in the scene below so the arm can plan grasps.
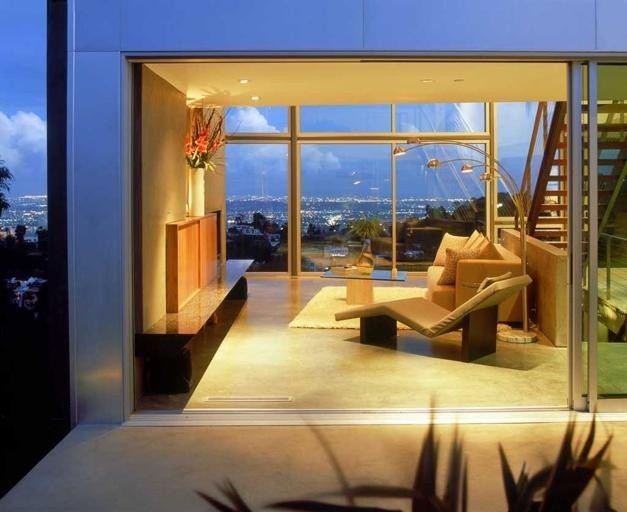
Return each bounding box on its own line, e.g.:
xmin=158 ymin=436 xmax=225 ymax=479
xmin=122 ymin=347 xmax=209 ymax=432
xmin=287 ymin=285 xmax=430 ymax=330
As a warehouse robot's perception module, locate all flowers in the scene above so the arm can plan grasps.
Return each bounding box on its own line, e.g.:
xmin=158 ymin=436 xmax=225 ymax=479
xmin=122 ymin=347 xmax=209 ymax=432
xmin=183 ymin=96 xmax=243 ymax=175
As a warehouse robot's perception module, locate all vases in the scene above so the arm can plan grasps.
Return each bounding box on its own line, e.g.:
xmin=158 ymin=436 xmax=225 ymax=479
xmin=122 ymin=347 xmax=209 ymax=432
xmin=187 ymin=168 xmax=205 ymax=218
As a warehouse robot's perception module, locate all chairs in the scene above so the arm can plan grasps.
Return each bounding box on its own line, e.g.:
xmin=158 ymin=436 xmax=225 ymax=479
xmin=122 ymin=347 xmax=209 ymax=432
xmin=334 ymin=274 xmax=533 ymax=363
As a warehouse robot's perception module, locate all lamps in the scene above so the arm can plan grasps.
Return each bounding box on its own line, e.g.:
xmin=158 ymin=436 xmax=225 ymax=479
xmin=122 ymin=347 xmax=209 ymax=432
xmin=390 ymin=141 xmax=537 ymax=344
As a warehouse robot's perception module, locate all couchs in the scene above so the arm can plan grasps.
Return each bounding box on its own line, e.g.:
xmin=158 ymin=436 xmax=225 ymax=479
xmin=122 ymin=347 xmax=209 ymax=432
xmin=425 ymin=229 xmax=527 ymax=322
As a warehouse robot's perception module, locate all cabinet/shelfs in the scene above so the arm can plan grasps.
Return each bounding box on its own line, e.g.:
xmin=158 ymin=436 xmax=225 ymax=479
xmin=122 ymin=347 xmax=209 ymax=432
xmin=166 ymin=217 xmax=200 ymax=313
xmin=200 ymin=211 xmax=217 ymax=292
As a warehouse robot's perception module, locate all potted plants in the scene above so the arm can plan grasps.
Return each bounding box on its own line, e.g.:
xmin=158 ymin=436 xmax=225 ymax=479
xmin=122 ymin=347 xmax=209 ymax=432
xmin=348 ymin=219 xmax=385 ymax=267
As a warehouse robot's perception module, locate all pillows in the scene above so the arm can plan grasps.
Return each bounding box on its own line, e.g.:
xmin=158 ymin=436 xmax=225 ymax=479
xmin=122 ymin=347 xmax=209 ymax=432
xmin=433 ymin=229 xmax=505 ymax=285
xmin=475 ymin=272 xmax=512 ymax=294
